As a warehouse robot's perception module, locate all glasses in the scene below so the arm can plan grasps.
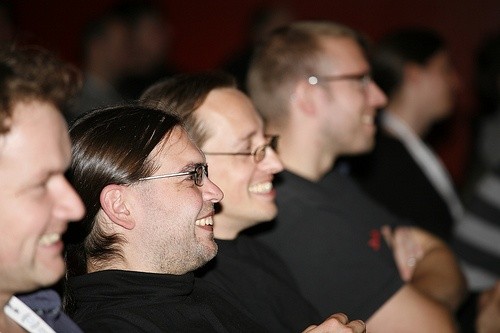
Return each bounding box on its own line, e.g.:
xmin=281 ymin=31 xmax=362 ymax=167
xmin=138 ymin=165 xmax=209 ymax=186
xmin=312 ymin=69 xmax=373 ymax=84
xmin=203 ymin=133 xmax=279 ymax=162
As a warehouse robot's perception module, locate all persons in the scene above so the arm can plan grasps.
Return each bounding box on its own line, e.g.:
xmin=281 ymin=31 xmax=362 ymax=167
xmin=0 ymin=0 xmax=500 ymax=333
